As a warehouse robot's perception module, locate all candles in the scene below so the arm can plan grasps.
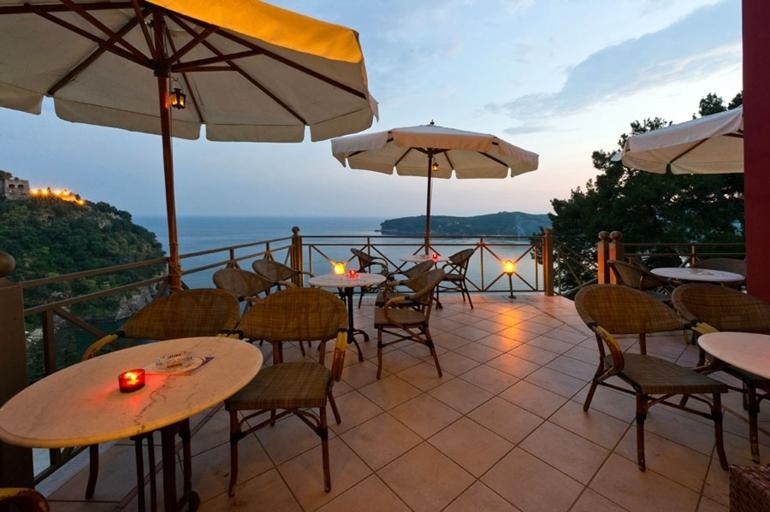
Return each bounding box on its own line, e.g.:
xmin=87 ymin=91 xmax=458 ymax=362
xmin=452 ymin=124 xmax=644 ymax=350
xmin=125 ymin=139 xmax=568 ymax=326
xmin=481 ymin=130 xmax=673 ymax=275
xmin=348 ymin=269 xmax=356 ymax=279
xmin=432 ymin=253 xmax=438 ymax=259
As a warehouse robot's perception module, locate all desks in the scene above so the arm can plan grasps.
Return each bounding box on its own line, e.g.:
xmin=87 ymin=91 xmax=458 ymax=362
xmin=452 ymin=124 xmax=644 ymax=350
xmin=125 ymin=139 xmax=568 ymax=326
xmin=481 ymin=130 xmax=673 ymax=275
xmin=697 ymin=330 xmax=770 ymax=464
xmin=0 ymin=335 xmax=264 ymax=512
xmin=650 ymin=267 xmax=745 ymax=295
xmin=309 ymin=272 xmax=387 ymax=361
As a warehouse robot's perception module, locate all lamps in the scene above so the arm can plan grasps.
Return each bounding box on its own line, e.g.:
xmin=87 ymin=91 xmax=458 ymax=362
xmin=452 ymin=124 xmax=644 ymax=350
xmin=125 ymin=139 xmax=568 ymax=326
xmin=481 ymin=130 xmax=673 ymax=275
xmin=503 ymin=259 xmax=516 ymax=298
xmin=168 ymin=72 xmax=187 ymax=110
xmin=432 ymin=156 xmax=440 ymax=172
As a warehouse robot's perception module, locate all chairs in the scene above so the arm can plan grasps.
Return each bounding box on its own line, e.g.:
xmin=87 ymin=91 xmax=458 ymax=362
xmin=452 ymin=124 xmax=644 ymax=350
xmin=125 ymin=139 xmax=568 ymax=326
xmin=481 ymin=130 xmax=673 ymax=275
xmin=223 ymin=286 xmax=351 ymax=496
xmin=0 ymin=488 xmax=50 ymax=511
xmin=375 ymin=269 xmax=446 ymax=378
xmin=574 ymin=283 xmax=732 ymax=473
xmin=213 ymin=268 xmax=308 ymax=358
xmin=661 ymin=283 xmax=770 ymax=409
xmin=607 ymin=259 xmax=668 ymax=291
xmin=375 ymin=259 xmax=434 ymax=330
xmin=435 ymin=248 xmax=475 ymax=310
xmin=82 ymin=288 xmax=240 ymax=499
xmin=351 ymin=247 xmax=388 ymax=309
xmin=252 ymin=259 xmax=316 ymax=294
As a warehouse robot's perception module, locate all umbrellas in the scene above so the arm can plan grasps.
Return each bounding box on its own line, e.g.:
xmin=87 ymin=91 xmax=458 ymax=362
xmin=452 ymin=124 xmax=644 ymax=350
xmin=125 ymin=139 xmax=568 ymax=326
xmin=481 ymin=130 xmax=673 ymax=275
xmin=0 ymin=1 xmax=378 ymax=293
xmin=621 ymin=105 xmax=745 ymax=176
xmin=328 ymin=120 xmax=540 ymax=257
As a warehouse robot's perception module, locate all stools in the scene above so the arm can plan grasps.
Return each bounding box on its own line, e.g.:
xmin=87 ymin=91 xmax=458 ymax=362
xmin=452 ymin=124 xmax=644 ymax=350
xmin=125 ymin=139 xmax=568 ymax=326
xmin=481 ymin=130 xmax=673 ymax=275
xmin=727 ymin=464 xmax=769 ymax=511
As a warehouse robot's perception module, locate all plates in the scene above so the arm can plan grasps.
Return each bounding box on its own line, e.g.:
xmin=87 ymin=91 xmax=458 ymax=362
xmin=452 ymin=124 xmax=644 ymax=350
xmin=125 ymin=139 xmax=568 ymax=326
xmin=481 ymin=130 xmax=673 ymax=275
xmin=145 ymin=358 xmax=202 ymax=374
